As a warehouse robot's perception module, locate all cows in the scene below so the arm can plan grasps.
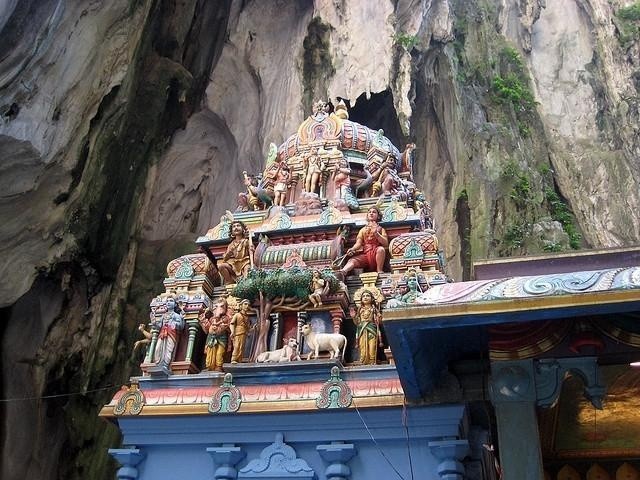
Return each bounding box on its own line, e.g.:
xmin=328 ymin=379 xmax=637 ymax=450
xmin=255 ymin=335 xmax=302 ymax=364
xmin=299 ymin=323 xmax=348 ymax=364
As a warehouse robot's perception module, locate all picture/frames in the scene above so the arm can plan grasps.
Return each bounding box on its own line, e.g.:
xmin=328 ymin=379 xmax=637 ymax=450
xmin=546 ymin=364 xmax=640 ymax=459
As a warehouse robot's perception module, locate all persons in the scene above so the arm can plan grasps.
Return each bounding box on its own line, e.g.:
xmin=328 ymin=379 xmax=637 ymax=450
xmin=339 ymin=207 xmax=388 ymax=275
xmin=154 ymin=295 xmax=186 ymax=369
xmin=239 ymin=147 xmax=394 ymax=206
xmin=230 ymin=299 xmax=251 ymax=364
xmin=352 ymin=291 xmax=381 ymax=364
xmin=218 ymin=220 xmax=250 ymax=285
xmin=309 ymin=270 xmax=325 ymax=308
xmin=401 ymin=273 xmax=421 ymax=301
